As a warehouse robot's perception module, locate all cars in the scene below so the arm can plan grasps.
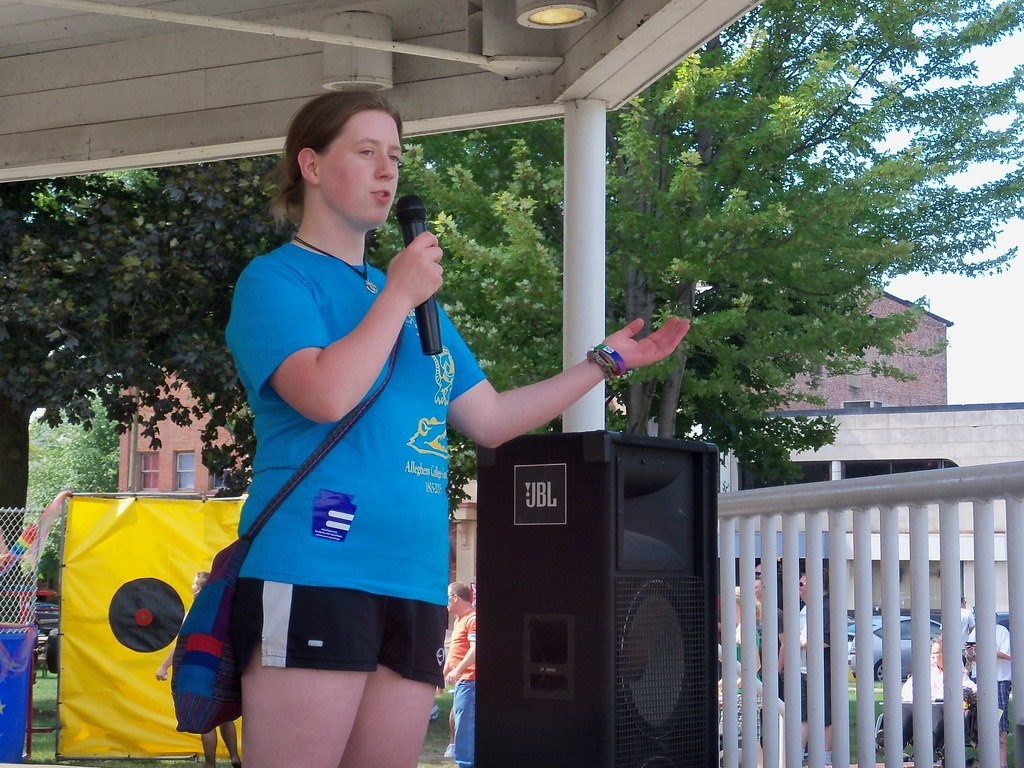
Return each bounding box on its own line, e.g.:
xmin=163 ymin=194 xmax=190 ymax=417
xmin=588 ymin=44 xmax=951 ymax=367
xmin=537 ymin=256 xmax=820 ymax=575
xmin=848 ymin=616 xmax=942 ymax=681
xmin=35 ymin=602 xmax=60 ymax=635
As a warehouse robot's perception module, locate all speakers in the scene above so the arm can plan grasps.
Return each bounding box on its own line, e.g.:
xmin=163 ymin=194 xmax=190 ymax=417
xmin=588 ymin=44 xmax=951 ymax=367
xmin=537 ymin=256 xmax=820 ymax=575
xmin=475 ymin=429 xmax=721 ymax=768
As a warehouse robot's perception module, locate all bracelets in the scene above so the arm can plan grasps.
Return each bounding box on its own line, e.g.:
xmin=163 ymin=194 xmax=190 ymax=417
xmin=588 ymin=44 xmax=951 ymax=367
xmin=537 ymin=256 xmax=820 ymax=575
xmin=586 ymin=344 xmax=626 ymax=381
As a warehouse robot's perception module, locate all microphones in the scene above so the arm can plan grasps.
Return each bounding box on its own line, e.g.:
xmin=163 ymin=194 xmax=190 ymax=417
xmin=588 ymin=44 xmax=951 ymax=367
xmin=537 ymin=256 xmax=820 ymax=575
xmin=396 ymin=194 xmax=442 ymax=356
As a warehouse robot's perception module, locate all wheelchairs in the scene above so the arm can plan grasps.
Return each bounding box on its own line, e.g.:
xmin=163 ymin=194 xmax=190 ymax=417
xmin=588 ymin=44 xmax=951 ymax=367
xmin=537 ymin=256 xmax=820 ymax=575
xmin=874 ymin=672 xmax=977 ymax=765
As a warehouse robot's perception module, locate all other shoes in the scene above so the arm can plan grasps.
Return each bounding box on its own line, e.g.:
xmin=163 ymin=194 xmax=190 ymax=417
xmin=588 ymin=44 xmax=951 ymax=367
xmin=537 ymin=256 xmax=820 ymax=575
xmin=444 ymin=743 xmax=455 ymax=759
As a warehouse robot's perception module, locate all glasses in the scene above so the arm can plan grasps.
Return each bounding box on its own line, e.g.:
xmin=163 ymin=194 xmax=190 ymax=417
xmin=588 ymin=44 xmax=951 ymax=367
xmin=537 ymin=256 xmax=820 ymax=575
xmin=798 ymin=582 xmax=808 ymax=587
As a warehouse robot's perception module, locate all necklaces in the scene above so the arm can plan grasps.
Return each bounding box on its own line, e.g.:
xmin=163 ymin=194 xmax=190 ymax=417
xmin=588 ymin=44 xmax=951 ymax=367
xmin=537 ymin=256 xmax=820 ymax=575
xmin=293 ymin=236 xmax=378 ymax=294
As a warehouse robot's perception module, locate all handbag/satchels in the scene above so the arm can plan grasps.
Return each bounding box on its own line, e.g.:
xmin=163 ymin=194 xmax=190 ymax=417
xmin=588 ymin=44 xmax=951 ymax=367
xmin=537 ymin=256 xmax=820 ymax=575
xmin=169 ymin=536 xmax=249 ymax=733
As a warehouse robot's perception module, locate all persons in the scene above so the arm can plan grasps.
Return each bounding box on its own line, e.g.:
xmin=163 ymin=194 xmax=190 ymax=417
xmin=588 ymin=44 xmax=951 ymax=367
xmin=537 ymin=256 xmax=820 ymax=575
xmin=939 ymin=597 xmax=976 ymax=650
xmin=155 ymin=571 xmax=241 ymax=768
xmin=876 ymin=639 xmax=977 ymax=762
xmin=964 ymin=623 xmax=1011 ymax=768
xmin=223 ymin=92 xmax=690 ymax=768
xmin=717 ymin=565 xmax=834 ymax=768
xmin=438 ymin=582 xmax=476 ymax=768
